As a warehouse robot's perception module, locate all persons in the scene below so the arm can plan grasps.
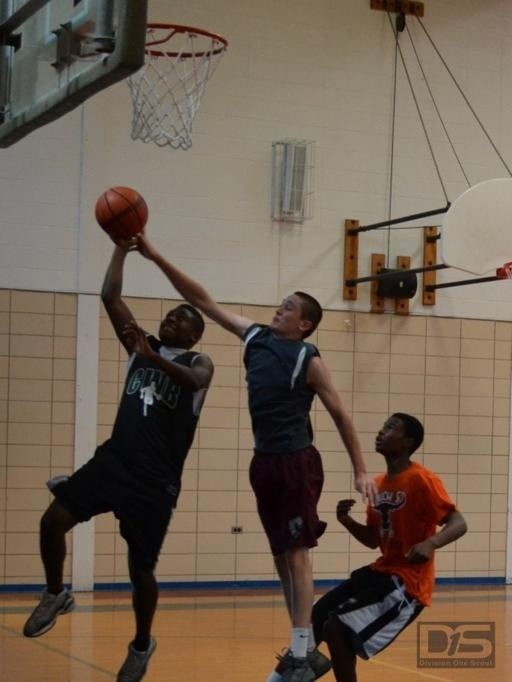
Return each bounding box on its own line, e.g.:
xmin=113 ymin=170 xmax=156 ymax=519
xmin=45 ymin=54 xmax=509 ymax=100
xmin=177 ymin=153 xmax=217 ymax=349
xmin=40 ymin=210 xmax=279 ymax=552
xmin=21 ymin=230 xmax=215 ymax=682
xmin=122 ymin=227 xmax=379 ymax=680
xmin=264 ymin=412 xmax=468 ymax=681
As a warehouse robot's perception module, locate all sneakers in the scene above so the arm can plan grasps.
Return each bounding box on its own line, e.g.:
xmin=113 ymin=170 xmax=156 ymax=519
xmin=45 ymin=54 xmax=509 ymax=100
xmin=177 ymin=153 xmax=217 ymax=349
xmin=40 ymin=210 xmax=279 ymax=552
xmin=23 ymin=586 xmax=74 ymax=637
xmin=277 ymin=646 xmax=331 ymax=682
xmin=117 ymin=636 xmax=156 ymax=682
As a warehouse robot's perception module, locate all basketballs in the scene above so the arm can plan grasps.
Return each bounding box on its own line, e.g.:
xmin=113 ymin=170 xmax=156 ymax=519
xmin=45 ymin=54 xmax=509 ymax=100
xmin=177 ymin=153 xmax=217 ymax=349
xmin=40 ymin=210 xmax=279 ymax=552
xmin=95 ymin=187 xmax=148 ymax=237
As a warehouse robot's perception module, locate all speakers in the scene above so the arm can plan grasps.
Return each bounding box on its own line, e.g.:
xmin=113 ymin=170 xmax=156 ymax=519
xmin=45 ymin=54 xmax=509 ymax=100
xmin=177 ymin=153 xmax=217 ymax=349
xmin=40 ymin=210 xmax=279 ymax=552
xmin=376 ymin=267 xmax=418 ymax=299
